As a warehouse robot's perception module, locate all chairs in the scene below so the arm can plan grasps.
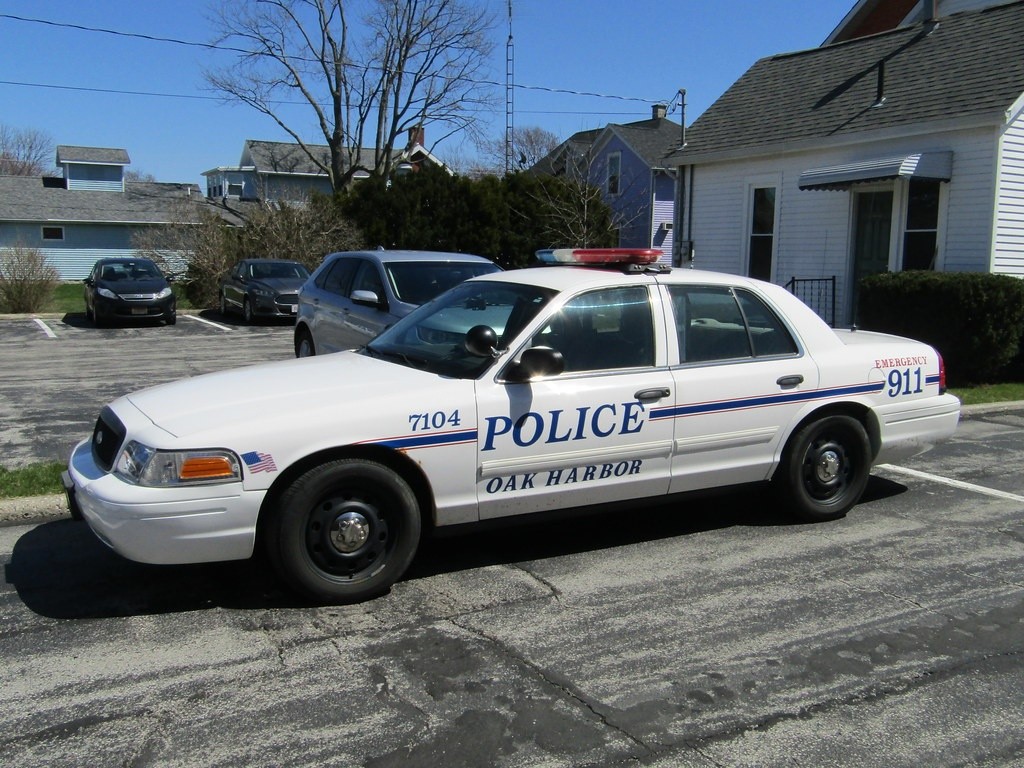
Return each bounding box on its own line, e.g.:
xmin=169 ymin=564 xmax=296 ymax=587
xmin=601 ymin=306 xmax=651 ymax=367
xmin=103 ymin=266 xmax=115 ymax=279
xmin=551 ymin=310 xmax=587 ymax=356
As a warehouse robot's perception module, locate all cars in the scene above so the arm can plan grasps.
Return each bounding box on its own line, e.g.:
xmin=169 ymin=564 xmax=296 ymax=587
xmin=59 ymin=246 xmax=962 ymax=606
xmin=82 ymin=257 xmax=177 ymax=329
xmin=218 ymin=258 xmax=311 ymax=325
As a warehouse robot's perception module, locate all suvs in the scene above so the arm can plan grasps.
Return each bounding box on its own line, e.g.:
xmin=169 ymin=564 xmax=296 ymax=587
xmin=293 ymin=246 xmax=553 ymax=375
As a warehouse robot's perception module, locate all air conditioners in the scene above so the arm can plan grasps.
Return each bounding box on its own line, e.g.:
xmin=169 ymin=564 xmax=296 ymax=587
xmin=662 ymin=222 xmax=673 ymax=231
